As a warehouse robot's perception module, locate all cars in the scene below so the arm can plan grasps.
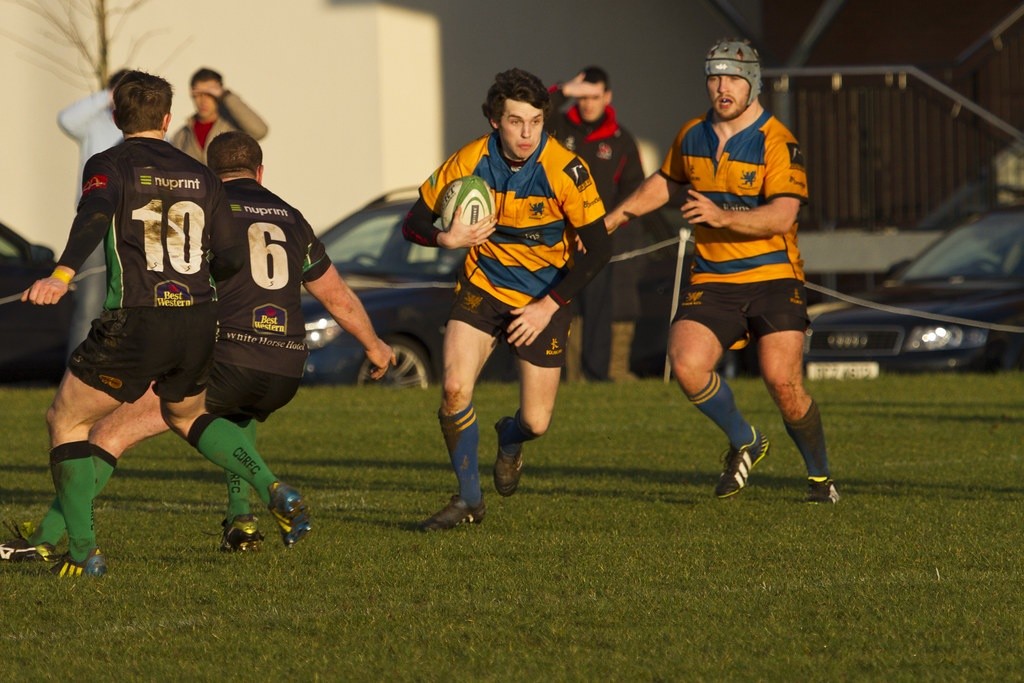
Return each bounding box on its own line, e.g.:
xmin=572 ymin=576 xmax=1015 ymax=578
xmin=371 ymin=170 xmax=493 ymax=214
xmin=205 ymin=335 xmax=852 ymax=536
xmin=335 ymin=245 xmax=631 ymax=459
xmin=810 ymin=202 xmax=1024 ymax=374
xmin=0 ymin=219 xmax=79 ymax=387
xmin=293 ymin=179 xmax=524 ymax=389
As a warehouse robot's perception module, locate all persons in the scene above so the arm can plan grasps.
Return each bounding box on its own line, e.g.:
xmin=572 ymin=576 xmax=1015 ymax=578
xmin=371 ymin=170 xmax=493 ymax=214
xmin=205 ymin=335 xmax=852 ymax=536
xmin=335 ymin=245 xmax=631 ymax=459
xmin=59 ymin=70 xmax=130 ymax=374
xmin=21 ymin=70 xmax=313 ymax=579
xmin=168 ymin=70 xmax=267 ymax=171
xmin=574 ymin=38 xmax=842 ymax=508
xmin=547 ymin=65 xmax=645 ymax=386
xmin=401 ymin=65 xmax=614 ymax=534
xmin=1 ymin=130 xmax=398 ymax=562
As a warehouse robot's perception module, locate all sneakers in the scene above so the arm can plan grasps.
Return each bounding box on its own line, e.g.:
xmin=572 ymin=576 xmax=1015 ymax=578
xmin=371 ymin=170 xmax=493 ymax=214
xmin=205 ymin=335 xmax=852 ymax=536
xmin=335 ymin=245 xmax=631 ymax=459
xmin=806 ymin=476 xmax=840 ymax=506
xmin=493 ymin=416 xmax=523 ymax=497
xmin=267 ymin=482 xmax=312 ymax=550
xmin=48 ymin=548 xmax=107 ymax=579
xmin=0 ymin=519 xmax=57 ymax=563
xmin=419 ymin=495 xmax=487 ymax=533
xmin=222 ymin=513 xmax=266 ymax=554
xmin=715 ymin=426 xmax=770 ymax=499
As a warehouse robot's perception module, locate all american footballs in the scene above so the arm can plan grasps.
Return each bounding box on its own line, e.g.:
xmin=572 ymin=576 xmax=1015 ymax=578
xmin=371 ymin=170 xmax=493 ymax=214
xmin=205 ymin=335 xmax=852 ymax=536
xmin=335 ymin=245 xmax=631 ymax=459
xmin=440 ymin=175 xmax=496 ymax=232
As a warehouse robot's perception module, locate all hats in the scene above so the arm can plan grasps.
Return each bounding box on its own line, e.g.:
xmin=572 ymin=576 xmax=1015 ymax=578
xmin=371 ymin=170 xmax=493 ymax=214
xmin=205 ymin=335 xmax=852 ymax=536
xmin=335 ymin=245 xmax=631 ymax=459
xmin=704 ymin=39 xmax=761 ymax=106
